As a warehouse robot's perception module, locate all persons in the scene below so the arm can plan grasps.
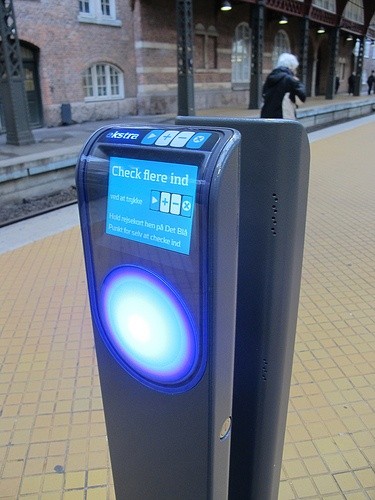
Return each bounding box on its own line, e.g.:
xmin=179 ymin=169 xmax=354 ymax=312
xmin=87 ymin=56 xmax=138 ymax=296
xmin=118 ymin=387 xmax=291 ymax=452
xmin=257 ymin=53 xmax=307 ymax=121
xmin=367 ymin=70 xmax=375 ymax=94
xmin=335 ymin=74 xmax=340 ymax=95
xmin=348 ymin=71 xmax=357 ymax=95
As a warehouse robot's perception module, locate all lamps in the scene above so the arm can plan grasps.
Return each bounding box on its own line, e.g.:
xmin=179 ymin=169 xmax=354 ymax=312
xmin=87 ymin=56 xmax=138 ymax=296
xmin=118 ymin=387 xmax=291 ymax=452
xmin=220 ymin=1 xmax=232 ymax=12
xmin=316 ymin=26 xmax=326 ymax=35
xmin=278 ymin=15 xmax=288 ymax=25
